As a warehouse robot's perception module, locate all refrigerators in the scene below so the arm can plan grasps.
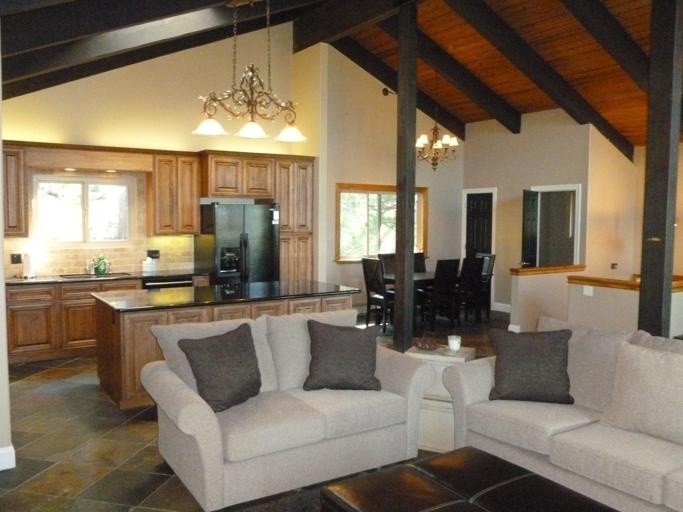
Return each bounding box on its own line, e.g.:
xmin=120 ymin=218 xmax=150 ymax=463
xmin=201 ymin=200 xmax=279 ymax=285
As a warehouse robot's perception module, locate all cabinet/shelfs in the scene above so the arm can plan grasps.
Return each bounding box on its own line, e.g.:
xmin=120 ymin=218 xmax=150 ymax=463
xmin=201 ymin=154 xmax=276 ymax=206
xmin=276 ymin=159 xmax=314 ymax=232
xmin=403 ymin=345 xmax=475 ymax=455
xmin=88 ymin=275 xmax=363 ymax=418
xmin=61 ymin=280 xmax=140 ymax=360
xmin=3 ymin=144 xmax=30 ymax=237
xmin=6 ymin=281 xmax=60 ymax=366
xmin=147 ymin=155 xmax=201 ymax=235
xmin=280 ymin=234 xmax=315 ymax=279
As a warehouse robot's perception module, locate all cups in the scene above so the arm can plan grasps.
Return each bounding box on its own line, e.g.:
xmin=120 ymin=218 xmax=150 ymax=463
xmin=448 ymin=336 xmax=461 ymax=352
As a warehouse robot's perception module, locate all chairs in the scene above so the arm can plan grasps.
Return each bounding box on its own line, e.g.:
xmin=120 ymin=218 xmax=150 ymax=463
xmin=379 ymin=253 xmax=395 ymax=321
xmin=417 ymin=258 xmax=460 ymax=331
xmin=414 ymin=253 xmax=428 ymax=319
xmin=361 ymin=258 xmax=394 ymax=332
xmin=381 ymin=271 xmax=493 ymax=326
xmin=450 ymin=258 xmax=485 ymax=323
xmin=458 ymin=255 xmax=497 ymax=320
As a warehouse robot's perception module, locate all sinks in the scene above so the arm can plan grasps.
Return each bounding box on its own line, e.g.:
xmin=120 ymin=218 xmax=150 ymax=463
xmin=59 ymin=272 xmax=137 ymax=280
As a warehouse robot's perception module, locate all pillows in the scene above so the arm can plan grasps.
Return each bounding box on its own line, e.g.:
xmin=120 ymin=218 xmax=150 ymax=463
xmin=628 ymin=331 xmax=683 ymax=356
xmin=178 ymin=324 xmax=262 ymax=412
xmin=602 ymin=342 xmax=683 ymax=443
xmin=536 ymin=315 xmax=622 ymax=414
xmin=151 ymin=315 xmax=278 ymax=401
xmin=268 ymin=310 xmax=359 ymax=391
xmin=304 ymin=319 xmax=383 ymax=392
xmin=490 ymin=328 xmax=574 ymax=404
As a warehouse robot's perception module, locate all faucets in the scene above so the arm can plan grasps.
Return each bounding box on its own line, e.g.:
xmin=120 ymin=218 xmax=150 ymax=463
xmin=89 ymin=256 xmax=104 ymax=274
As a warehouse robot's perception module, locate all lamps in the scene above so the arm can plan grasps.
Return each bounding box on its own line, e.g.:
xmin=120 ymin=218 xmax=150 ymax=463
xmin=191 ymin=0 xmax=305 ymax=144
xmin=416 ymin=70 xmax=459 ymax=172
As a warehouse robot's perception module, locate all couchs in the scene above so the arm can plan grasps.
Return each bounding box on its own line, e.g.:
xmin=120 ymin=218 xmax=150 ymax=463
xmin=140 ymin=345 xmax=434 ymax=512
xmin=442 ymin=347 xmax=683 ymax=512
